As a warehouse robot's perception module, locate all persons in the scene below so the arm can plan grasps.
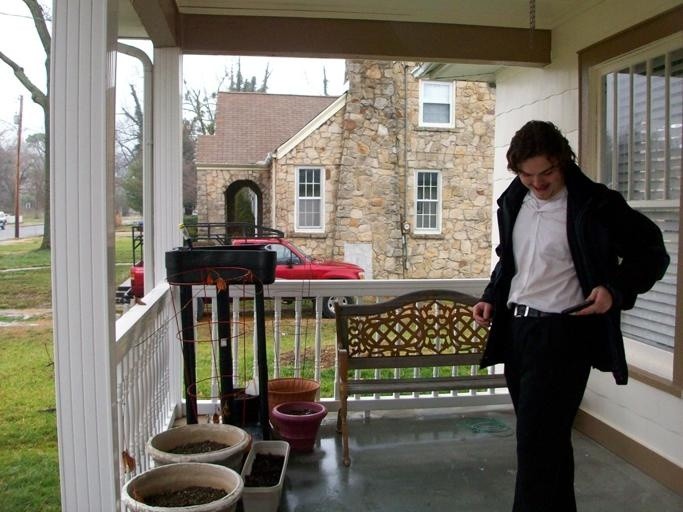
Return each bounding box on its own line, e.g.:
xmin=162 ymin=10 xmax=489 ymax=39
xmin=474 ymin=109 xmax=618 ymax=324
xmin=469 ymin=119 xmax=672 ymax=512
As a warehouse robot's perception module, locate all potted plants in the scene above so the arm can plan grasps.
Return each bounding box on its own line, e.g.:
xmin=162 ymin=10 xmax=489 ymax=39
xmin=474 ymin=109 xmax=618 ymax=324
xmin=119 ymin=377 xmax=328 ymax=512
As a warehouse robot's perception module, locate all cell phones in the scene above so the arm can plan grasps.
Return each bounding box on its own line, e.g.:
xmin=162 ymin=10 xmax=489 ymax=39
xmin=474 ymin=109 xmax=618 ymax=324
xmin=561 ymin=300 xmax=595 ymax=316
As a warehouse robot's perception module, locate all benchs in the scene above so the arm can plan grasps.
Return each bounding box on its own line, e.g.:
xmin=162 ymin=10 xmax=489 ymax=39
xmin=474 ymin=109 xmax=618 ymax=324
xmin=334 ymin=288 xmax=509 ymax=466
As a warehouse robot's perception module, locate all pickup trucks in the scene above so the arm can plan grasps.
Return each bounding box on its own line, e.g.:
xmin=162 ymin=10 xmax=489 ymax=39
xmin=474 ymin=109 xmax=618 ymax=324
xmin=130 ymin=237 xmax=364 ymax=318
